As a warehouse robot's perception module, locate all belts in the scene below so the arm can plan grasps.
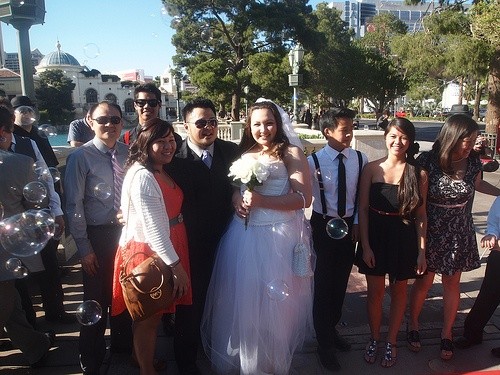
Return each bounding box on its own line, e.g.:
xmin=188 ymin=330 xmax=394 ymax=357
xmin=428 ymin=201 xmax=468 ymax=208
xmin=370 ymin=206 xmax=407 ymax=216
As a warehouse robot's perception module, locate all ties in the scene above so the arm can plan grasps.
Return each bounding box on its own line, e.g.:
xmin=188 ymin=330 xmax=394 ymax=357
xmin=106 ymin=148 xmax=123 ymax=212
xmin=202 ymin=151 xmax=212 ymax=168
xmin=336 ymin=153 xmax=346 ymax=218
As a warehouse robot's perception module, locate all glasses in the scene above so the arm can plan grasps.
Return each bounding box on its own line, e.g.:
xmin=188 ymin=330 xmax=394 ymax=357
xmin=92 ymin=116 xmax=121 ymax=124
xmin=135 ymin=98 xmax=160 ymax=107
xmin=188 ymin=117 xmax=219 ymax=128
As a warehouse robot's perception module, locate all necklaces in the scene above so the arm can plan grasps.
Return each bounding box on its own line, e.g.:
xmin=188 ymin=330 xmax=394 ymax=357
xmin=452 ymin=157 xmax=463 ymax=162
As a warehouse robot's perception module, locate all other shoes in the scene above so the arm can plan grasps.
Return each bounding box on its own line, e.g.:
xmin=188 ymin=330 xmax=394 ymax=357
xmin=60 ymin=314 xmax=77 ymax=322
xmin=492 ymin=346 xmax=500 ymax=356
xmin=335 ymin=335 xmax=351 ymax=351
xmin=33 ymin=351 xmax=49 ymax=369
xmin=128 ymin=352 xmax=168 ymax=370
xmin=318 ymin=345 xmax=340 ymax=371
xmin=178 ymin=363 xmax=202 ymax=375
xmin=84 ymin=366 xmax=100 ymax=375
xmin=452 ymin=335 xmax=482 ymax=348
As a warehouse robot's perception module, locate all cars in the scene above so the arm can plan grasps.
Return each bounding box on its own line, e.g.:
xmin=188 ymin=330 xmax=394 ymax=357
xmin=478 ymin=109 xmax=487 ymax=122
xmin=395 ymin=110 xmax=407 ymax=116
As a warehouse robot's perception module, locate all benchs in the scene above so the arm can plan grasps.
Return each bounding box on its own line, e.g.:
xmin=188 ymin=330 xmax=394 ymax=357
xmin=364 ymin=124 xmax=379 ymax=129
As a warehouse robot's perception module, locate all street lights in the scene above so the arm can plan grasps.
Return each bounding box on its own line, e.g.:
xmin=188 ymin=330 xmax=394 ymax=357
xmin=244 ymin=86 xmax=250 ymax=121
xmin=173 ymin=74 xmax=181 ymax=122
xmin=287 ymin=42 xmax=304 ymax=126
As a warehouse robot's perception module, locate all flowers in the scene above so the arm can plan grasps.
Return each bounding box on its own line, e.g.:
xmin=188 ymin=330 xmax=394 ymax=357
xmin=227 ymin=156 xmax=270 ymax=231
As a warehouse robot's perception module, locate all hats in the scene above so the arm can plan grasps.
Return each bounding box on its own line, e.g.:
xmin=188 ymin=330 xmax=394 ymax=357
xmin=443 ymin=104 xmax=474 ymax=116
xmin=10 ymin=96 xmax=35 ymax=109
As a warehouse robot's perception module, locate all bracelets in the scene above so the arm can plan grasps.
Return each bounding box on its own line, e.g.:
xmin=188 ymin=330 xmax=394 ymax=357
xmin=170 ymin=260 xmax=181 ymax=268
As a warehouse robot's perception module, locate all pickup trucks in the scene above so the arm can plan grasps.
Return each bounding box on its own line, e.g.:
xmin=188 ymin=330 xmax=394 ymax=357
xmin=442 ymin=104 xmax=470 ymax=118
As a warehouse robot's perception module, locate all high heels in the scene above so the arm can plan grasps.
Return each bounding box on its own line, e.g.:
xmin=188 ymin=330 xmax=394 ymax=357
xmin=406 ymin=322 xmax=421 ymax=352
xmin=364 ymin=335 xmax=379 ymax=363
xmin=440 ymin=332 xmax=453 ymax=359
xmin=381 ymin=339 xmax=396 ymax=368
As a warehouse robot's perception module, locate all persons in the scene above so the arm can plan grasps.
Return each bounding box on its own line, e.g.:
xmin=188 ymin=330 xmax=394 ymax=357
xmin=355 ymin=117 xmax=427 ymax=368
xmin=199 ymin=97 xmax=313 ymax=375
xmin=306 ymin=106 xmax=369 ymax=371
xmin=453 ymin=196 xmax=500 ymax=357
xmin=302 ymin=108 xmax=390 ymax=131
xmin=0 ymin=83 xmax=239 ymax=375
xmin=408 ymin=114 xmax=500 ymax=359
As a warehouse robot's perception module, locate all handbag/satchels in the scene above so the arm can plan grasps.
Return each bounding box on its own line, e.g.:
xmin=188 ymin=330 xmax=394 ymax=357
xmin=294 ymin=190 xmax=313 ymax=278
xmin=120 ymin=251 xmax=176 ymax=320
